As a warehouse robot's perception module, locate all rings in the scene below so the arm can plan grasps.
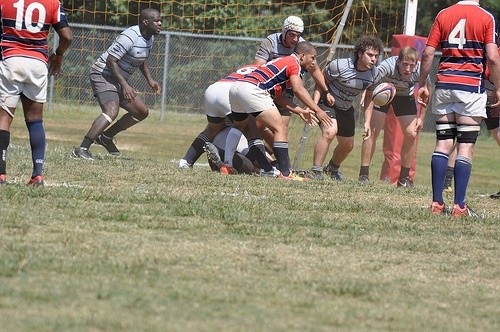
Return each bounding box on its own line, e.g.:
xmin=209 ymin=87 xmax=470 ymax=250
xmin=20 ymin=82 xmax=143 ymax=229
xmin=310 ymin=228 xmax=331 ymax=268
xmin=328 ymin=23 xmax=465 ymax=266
xmin=310 ymin=121 xmax=312 ymax=123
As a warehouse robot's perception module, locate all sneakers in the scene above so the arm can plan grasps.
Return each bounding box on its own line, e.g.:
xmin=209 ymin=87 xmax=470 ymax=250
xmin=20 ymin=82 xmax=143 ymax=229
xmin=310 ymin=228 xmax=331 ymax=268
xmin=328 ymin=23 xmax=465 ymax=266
xmin=490 ymin=191 xmax=500 ymax=199
xmin=279 ymin=170 xmax=305 ymax=181
xmin=323 ymin=165 xmax=342 ymax=180
xmin=70 ymin=146 xmax=94 ymax=161
xmin=270 ymin=160 xmax=281 ymax=170
xmin=311 ymin=168 xmax=325 ymax=180
xmin=26 ymin=175 xmax=44 ymax=187
xmin=431 ymin=201 xmax=451 ymax=215
xmin=443 ymin=182 xmax=453 ymax=194
xmin=179 ymin=159 xmax=192 ymax=170
xmin=397 ymin=180 xmax=414 ymax=190
xmin=94 ymin=133 xmax=120 ymax=155
xmin=261 ymin=166 xmax=281 ymax=177
xmin=204 ymin=141 xmax=221 ymax=163
xmin=220 ymin=164 xmax=238 ymax=175
xmin=451 ymin=204 xmax=478 ymax=217
xmin=0 ymin=174 xmax=6 ymax=183
xmin=358 ymin=175 xmax=370 ymax=186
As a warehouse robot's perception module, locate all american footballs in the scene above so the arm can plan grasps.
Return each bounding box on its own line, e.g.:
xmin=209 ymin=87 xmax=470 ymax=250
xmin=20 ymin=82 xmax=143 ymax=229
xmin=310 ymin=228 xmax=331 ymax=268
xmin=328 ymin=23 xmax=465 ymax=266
xmin=372 ymin=82 xmax=397 ymax=107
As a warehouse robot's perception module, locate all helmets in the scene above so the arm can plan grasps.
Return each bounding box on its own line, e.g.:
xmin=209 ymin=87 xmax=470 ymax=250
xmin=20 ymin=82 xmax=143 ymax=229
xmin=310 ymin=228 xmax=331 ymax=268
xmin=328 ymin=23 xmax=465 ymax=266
xmin=282 ymin=15 xmax=304 ymax=40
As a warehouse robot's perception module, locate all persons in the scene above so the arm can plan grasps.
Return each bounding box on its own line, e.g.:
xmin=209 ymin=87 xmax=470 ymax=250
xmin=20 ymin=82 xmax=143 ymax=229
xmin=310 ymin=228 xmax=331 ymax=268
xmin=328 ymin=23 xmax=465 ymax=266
xmin=0 ymin=0 xmax=72 ymax=184
xmin=80 ymin=0 xmax=500 ymax=218
